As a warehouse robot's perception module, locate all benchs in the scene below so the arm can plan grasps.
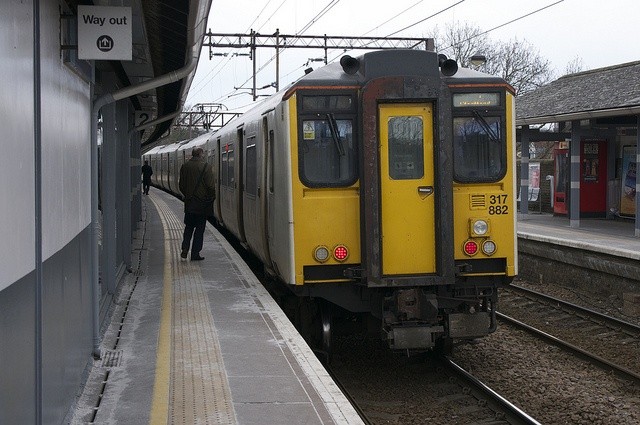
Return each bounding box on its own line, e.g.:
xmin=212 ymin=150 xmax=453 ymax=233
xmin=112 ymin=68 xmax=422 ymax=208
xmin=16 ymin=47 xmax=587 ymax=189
xmin=517 ymin=187 xmax=541 ymax=214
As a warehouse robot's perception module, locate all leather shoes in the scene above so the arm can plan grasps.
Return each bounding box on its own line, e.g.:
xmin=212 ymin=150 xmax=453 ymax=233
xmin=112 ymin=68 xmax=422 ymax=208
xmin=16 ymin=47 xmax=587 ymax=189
xmin=191 ymin=254 xmax=205 ymax=261
xmin=180 ymin=252 xmax=187 ymax=259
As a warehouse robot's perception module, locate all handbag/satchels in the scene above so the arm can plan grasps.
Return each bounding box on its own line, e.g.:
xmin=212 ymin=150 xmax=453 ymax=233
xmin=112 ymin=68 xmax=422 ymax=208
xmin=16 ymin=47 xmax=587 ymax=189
xmin=191 ymin=198 xmax=210 ymax=217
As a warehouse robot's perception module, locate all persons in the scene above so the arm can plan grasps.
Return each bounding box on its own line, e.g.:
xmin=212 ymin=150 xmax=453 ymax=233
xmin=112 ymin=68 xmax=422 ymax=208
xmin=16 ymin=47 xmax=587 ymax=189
xmin=141 ymin=160 xmax=153 ymax=195
xmin=179 ymin=148 xmax=216 ymax=261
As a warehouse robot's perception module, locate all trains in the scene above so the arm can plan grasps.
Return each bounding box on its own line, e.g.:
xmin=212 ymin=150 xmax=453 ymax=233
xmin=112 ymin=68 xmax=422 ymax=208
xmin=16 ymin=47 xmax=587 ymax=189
xmin=141 ymin=50 xmax=519 ymax=357
